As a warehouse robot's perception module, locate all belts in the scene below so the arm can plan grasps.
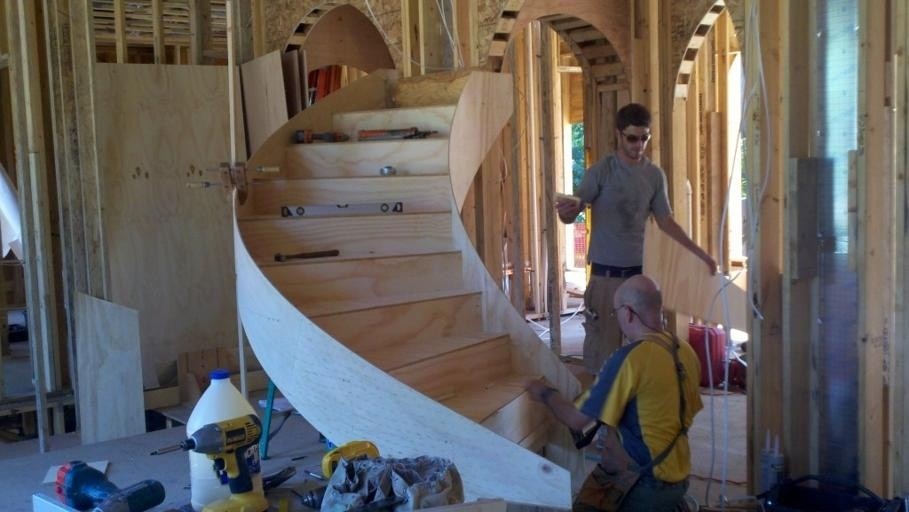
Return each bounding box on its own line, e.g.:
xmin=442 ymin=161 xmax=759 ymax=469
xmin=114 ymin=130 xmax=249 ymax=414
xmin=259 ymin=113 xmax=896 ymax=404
xmin=641 ymin=473 xmax=690 ymax=491
xmin=592 ymin=263 xmax=645 ymax=278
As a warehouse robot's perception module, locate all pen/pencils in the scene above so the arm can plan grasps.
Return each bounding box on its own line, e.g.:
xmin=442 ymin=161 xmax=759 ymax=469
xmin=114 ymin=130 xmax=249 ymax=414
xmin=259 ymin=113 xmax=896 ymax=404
xmin=291 ymin=489 xmax=302 ymax=498
xmin=304 ymin=470 xmax=325 ymax=480
xmin=292 ymin=455 xmax=308 ymax=461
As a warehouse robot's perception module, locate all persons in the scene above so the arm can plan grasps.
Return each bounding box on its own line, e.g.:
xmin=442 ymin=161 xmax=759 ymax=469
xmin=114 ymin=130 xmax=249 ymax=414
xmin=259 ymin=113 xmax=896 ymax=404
xmin=523 ymin=272 xmax=706 ymax=510
xmin=551 ymin=101 xmax=720 ymax=380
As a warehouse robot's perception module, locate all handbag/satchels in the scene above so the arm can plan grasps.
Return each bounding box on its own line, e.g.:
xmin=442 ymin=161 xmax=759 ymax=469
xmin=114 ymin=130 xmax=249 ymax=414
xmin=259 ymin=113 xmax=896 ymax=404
xmin=571 ymin=447 xmax=640 ymax=511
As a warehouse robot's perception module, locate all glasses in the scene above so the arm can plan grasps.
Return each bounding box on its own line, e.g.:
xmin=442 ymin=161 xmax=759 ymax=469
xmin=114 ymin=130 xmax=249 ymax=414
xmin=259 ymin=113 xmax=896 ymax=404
xmin=619 ymin=129 xmax=651 ymax=143
xmin=607 ymin=306 xmax=623 ymax=321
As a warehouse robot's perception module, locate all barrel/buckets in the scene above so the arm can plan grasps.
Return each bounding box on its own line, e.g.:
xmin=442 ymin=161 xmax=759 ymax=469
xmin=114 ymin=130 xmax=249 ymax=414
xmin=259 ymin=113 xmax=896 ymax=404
xmin=186 ymin=370 xmax=263 ymax=512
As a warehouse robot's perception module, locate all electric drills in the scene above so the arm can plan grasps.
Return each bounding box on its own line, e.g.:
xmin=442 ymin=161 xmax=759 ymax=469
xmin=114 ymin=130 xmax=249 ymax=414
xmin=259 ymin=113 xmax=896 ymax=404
xmin=149 ymin=414 xmax=270 ymax=512
xmin=56 ymin=460 xmax=165 ymax=512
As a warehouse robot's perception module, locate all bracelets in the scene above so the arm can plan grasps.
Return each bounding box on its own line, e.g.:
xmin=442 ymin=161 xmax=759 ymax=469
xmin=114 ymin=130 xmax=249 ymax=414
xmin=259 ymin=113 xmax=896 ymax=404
xmin=542 ymin=387 xmax=560 ymax=405
